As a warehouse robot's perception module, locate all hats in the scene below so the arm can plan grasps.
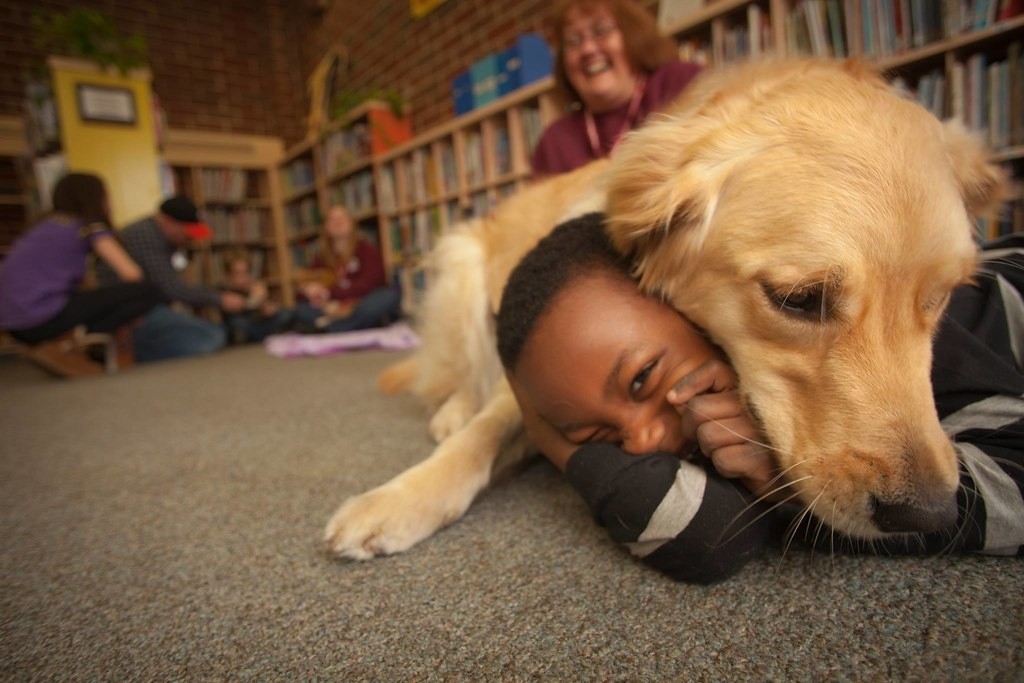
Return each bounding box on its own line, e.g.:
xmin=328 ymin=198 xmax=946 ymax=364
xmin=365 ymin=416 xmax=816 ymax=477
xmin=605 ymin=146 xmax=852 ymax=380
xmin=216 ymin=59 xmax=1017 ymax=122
xmin=159 ymin=196 xmax=215 ymax=242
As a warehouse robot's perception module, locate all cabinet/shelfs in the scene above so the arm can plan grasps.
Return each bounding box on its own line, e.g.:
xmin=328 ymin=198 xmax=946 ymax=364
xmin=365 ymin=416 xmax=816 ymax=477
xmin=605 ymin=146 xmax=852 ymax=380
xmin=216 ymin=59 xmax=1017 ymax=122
xmin=0 ymin=1 xmax=1024 ymax=352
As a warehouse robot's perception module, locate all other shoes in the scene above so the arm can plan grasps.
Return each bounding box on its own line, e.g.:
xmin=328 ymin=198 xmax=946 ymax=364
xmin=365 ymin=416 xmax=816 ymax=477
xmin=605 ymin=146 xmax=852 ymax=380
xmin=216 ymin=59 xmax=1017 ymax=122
xmin=0 ymin=330 xmax=26 ymax=354
xmin=31 ymin=340 xmax=105 ymax=382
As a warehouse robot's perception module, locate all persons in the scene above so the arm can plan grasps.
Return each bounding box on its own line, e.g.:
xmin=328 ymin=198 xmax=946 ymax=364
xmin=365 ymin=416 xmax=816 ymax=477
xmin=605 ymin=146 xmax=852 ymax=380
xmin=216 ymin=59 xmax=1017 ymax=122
xmin=220 ymin=251 xmax=291 ymax=345
xmin=0 ymin=171 xmax=147 ymax=377
xmin=291 ymin=205 xmax=402 ymax=333
xmin=93 ymin=194 xmax=243 ymax=363
xmin=497 ymin=232 xmax=1023 ymax=585
xmin=533 ymin=0 xmax=708 ymax=180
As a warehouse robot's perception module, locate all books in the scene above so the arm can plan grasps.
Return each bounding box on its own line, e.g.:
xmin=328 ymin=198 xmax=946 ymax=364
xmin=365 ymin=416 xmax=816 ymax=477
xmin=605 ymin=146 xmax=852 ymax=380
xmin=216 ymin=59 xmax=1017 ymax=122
xmin=680 ymin=0 xmax=1024 ymax=239
xmin=185 ymin=167 xmax=279 ymax=278
xmin=281 ymin=108 xmax=542 ymax=304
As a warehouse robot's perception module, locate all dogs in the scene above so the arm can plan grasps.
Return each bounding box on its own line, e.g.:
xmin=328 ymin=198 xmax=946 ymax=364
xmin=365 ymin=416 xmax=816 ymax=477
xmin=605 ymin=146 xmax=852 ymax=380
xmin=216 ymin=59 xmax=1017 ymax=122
xmin=320 ymin=57 xmax=1004 ymax=561
